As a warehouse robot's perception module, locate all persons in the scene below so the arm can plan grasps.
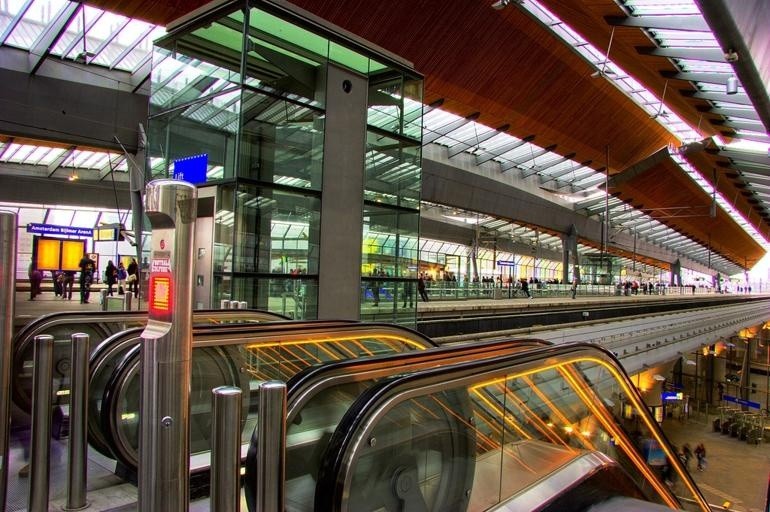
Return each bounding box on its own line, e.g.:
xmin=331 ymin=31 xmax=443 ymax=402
xmin=463 ymin=274 xmax=563 ymax=299
xmin=572 ymin=278 xmax=578 ymax=299
xmin=737 ymin=285 xmax=752 ymax=294
xmin=268 ymin=265 xmax=307 ymax=292
xmin=617 ymin=280 xmax=666 ymax=297
xmin=418 ymin=271 xmax=433 ymax=302
xmin=692 ymin=444 xmax=707 ymax=474
xmin=681 ymin=442 xmax=694 ymax=469
xmin=443 ymin=271 xmax=457 ymax=294
xmin=26 ymin=254 xmax=150 ymax=304
xmin=404 ymin=271 xmax=417 ymax=308
xmin=369 ymin=267 xmax=389 ymax=306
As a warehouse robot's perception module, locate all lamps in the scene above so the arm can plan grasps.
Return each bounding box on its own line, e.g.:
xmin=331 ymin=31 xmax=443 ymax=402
xmin=725 ymin=50 xmax=740 ymax=95
